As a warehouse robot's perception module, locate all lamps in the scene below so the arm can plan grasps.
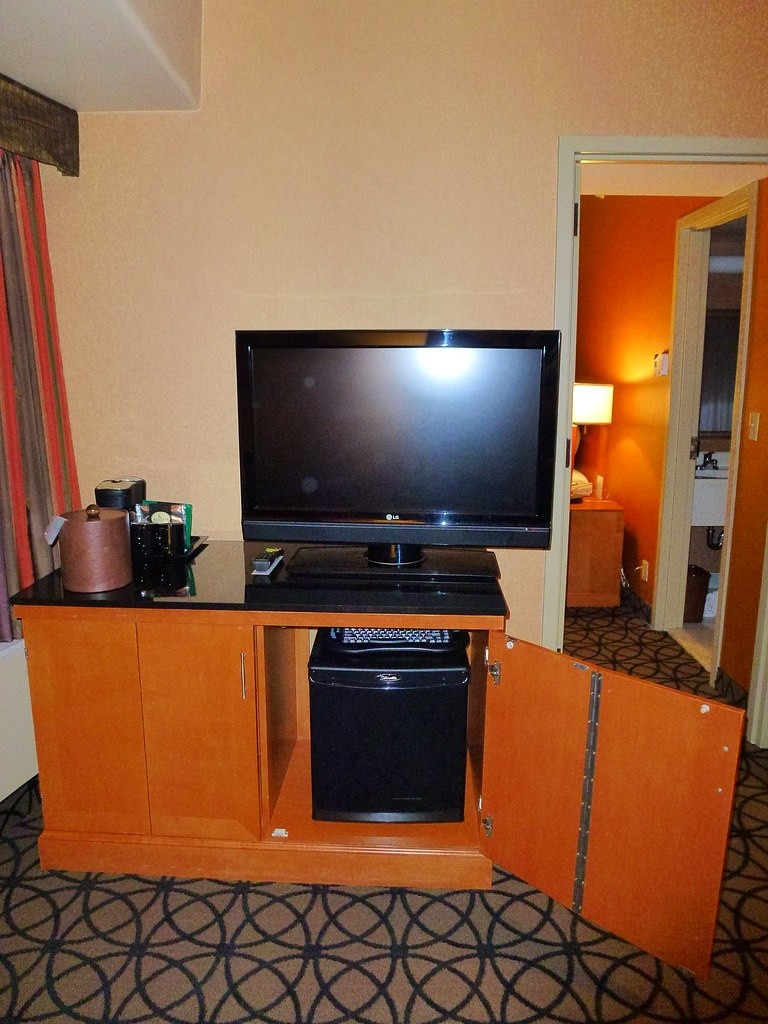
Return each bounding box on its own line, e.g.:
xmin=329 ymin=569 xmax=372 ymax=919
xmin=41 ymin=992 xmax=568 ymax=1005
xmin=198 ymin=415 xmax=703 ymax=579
xmin=572 ymin=382 xmax=614 ymax=434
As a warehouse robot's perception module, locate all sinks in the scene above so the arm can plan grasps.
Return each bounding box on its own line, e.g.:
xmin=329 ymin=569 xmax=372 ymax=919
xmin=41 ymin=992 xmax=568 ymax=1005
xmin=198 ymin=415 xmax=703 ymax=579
xmin=693 ymin=469 xmax=730 ymax=527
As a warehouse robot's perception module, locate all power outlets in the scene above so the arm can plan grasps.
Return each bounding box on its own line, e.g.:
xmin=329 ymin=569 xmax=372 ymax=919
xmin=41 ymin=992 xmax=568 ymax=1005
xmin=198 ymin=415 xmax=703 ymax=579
xmin=640 ymin=559 xmax=649 ymax=581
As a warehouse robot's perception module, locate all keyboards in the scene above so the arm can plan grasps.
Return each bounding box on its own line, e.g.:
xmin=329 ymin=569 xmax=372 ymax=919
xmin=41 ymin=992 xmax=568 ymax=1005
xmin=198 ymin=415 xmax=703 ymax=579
xmin=327 ymin=627 xmax=471 ymax=657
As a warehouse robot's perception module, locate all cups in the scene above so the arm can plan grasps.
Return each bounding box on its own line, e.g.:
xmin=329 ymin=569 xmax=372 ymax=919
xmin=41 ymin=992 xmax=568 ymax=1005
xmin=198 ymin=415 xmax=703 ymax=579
xmin=131 ymin=522 xmax=188 ymax=594
xmin=56 ymin=507 xmax=133 ymax=594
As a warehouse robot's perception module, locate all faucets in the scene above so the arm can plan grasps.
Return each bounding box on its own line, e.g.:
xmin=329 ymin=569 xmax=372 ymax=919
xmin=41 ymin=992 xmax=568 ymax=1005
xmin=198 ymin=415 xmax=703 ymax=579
xmin=703 ymin=451 xmax=718 ymax=467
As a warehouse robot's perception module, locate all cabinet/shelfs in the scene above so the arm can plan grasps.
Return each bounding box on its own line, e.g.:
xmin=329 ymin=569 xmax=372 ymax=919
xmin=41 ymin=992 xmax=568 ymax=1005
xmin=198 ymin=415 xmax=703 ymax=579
xmin=7 ymin=538 xmax=747 ymax=982
xmin=689 ymin=479 xmax=729 ymax=573
xmin=566 ymin=497 xmax=624 ymax=608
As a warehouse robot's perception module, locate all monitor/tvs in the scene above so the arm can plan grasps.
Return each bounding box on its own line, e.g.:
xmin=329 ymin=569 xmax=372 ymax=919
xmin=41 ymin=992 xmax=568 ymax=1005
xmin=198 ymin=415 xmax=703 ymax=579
xmin=231 ymin=330 xmax=562 ymax=583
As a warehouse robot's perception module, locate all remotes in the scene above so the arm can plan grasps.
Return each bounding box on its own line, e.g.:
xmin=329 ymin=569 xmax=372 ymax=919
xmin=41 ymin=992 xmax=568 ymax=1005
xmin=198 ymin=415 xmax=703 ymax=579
xmin=251 ymin=546 xmax=284 ymax=572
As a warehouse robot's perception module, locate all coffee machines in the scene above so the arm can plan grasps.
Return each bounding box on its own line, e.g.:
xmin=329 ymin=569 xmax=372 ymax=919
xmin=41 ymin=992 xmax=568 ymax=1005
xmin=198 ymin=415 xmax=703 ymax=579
xmin=94 ymin=475 xmax=146 ymax=524
